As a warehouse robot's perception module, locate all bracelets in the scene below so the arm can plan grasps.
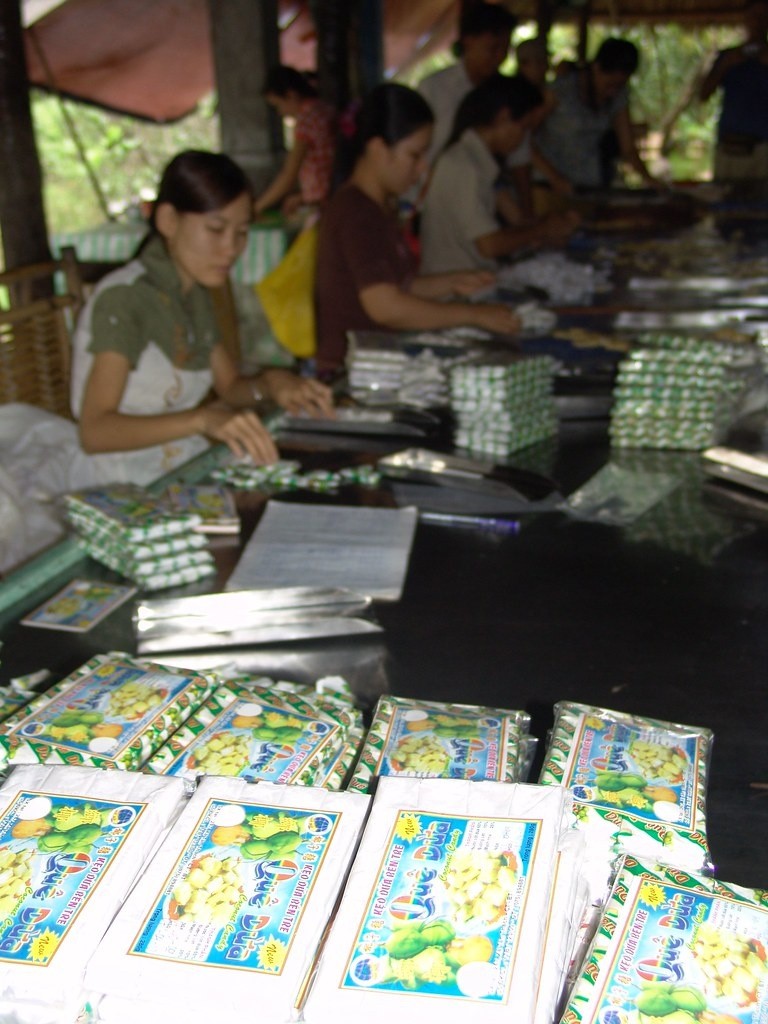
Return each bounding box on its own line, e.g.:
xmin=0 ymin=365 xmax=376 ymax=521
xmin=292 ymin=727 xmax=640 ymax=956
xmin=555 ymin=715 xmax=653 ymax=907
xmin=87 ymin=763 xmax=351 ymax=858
xmin=249 ymin=373 xmax=265 ymax=403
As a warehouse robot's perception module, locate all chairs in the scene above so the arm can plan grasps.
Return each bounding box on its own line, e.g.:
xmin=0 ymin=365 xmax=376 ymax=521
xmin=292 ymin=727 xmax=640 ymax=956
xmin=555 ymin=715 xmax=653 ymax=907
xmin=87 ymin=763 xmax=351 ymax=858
xmin=0 ymin=245 xmax=86 ymax=424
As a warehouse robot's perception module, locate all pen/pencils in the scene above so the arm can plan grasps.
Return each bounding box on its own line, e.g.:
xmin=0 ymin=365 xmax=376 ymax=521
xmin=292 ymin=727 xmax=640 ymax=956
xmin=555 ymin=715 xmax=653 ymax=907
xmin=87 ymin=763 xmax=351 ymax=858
xmin=417 ymin=511 xmax=522 ymax=531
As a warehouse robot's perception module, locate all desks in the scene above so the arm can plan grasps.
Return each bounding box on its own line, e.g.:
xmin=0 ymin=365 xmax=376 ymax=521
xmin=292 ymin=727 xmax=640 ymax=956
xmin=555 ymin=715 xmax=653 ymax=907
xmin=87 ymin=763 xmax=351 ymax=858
xmin=53 ymin=208 xmax=287 ymax=336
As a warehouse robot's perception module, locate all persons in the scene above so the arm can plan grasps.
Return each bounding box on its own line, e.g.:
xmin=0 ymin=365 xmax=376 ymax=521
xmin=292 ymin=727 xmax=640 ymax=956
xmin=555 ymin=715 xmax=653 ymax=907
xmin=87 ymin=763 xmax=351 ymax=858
xmin=252 ymin=66 xmax=335 ymax=219
xmin=310 ymin=77 xmax=523 ymax=390
xmin=71 ymin=149 xmax=333 ymax=463
xmin=411 ymin=1 xmax=767 ymax=199
xmin=418 ymin=79 xmax=572 ymax=308
